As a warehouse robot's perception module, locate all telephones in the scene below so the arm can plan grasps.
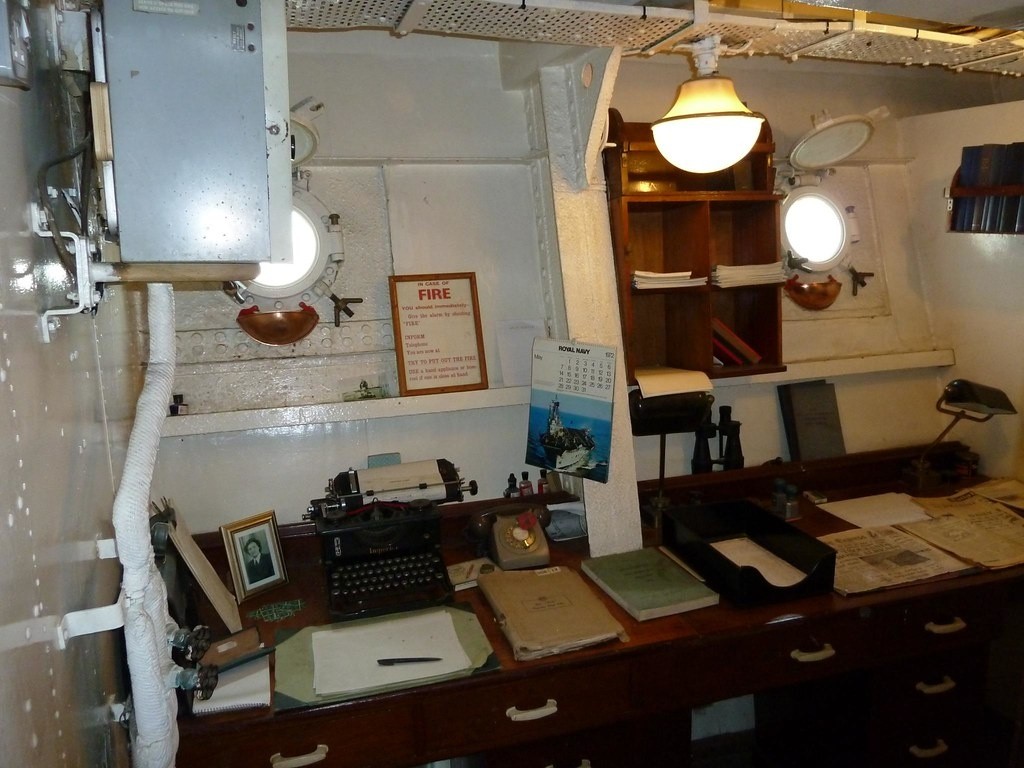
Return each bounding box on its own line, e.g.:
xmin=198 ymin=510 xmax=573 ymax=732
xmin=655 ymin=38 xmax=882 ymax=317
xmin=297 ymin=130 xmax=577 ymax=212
xmin=474 ymin=497 xmax=554 ymax=571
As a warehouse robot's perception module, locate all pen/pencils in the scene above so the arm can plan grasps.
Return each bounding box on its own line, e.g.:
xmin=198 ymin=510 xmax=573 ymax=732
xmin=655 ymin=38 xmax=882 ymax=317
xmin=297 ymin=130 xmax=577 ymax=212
xmin=376 ymin=657 xmax=443 ymax=666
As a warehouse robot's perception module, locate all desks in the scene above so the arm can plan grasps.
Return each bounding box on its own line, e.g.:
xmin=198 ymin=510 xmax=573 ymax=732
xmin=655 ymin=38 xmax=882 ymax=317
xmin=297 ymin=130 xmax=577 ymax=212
xmin=160 ymin=440 xmax=1024 ymax=768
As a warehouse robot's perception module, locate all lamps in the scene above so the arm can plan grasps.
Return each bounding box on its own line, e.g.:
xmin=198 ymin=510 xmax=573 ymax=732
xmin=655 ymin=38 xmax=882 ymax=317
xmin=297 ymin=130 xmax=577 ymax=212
xmin=650 ymin=35 xmax=765 ymax=174
xmin=628 ymin=389 xmax=710 ymax=529
xmin=897 ymin=380 xmax=1017 ymax=492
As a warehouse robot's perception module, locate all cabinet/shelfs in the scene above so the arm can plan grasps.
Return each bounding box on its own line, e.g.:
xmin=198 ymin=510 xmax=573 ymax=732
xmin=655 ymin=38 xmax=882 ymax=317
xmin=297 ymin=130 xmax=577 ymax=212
xmin=601 ymin=106 xmax=787 ymax=384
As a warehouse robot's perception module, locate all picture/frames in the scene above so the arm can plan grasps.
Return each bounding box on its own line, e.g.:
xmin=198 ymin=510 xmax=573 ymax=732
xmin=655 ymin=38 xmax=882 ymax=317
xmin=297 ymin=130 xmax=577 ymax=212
xmin=219 ymin=508 xmax=289 ymax=606
